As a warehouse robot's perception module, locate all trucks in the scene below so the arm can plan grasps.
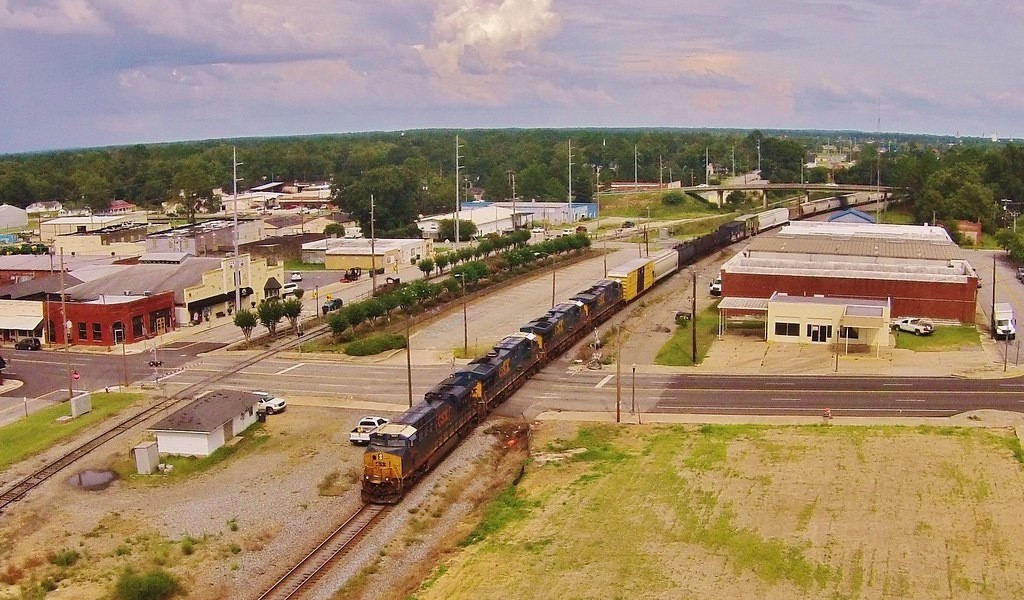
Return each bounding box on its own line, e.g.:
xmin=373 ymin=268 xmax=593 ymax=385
xmin=991 ymin=303 xmax=1016 ymax=339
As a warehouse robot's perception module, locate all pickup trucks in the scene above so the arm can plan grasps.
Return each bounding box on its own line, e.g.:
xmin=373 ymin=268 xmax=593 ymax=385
xmin=350 ymin=416 xmax=389 ymax=444
xmin=893 ymin=318 xmax=936 ymax=336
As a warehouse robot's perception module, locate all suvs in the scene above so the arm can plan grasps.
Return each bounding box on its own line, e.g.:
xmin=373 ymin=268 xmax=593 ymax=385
xmin=576 ymin=226 xmax=587 ymax=232
xmin=710 ymin=278 xmax=721 ymax=295
xmin=15 ymin=338 xmax=41 ymax=350
xmin=284 ymin=283 xmax=299 ymax=294
xmin=291 ymin=271 xmax=303 ymax=282
xmin=252 ymin=392 xmax=286 ymax=415
xmin=322 ymin=298 xmax=342 ymax=311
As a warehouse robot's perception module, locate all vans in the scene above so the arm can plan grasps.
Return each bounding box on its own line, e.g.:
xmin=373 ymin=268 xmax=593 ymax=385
xmin=563 ymin=229 xmax=574 ymax=236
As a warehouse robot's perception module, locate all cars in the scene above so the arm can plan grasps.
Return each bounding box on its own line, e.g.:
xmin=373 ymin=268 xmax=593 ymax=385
xmin=1016 ymin=268 xmax=1024 ymax=284
xmin=977 ymin=274 xmax=982 ymax=288
xmin=533 ymin=227 xmax=543 ymax=233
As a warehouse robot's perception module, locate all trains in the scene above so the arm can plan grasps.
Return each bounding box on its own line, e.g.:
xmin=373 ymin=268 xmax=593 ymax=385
xmin=360 ymin=192 xmax=893 ymax=505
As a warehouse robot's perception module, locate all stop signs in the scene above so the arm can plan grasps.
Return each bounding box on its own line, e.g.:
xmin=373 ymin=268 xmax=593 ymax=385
xmin=73 ymin=372 xmax=79 ymax=380
